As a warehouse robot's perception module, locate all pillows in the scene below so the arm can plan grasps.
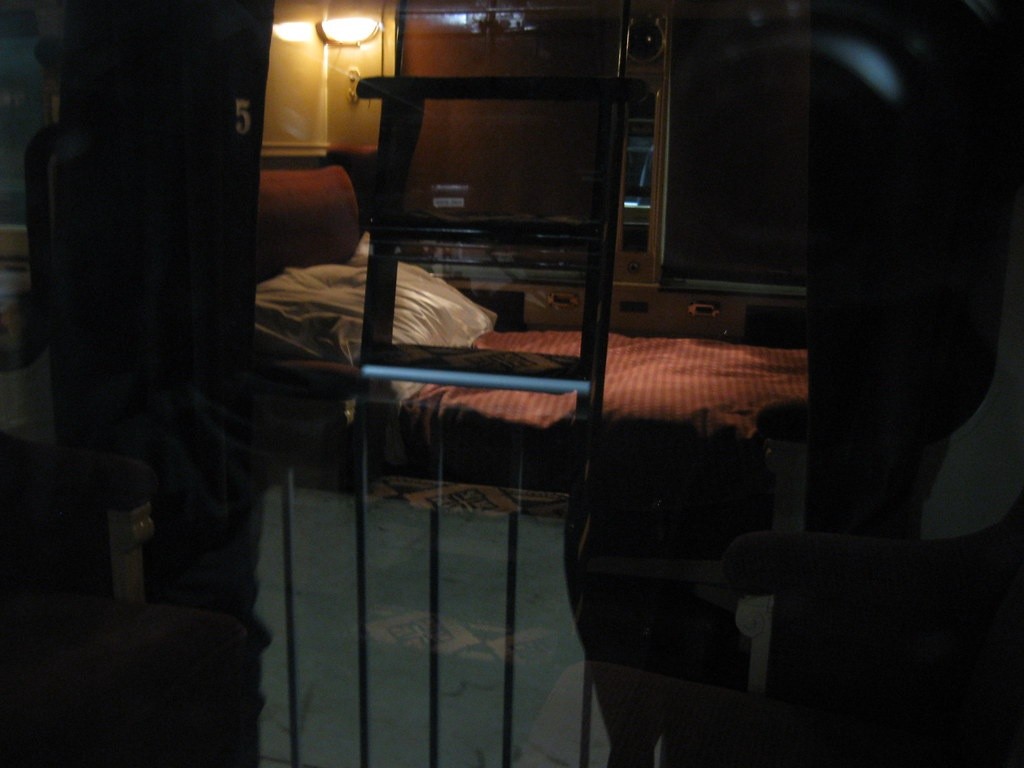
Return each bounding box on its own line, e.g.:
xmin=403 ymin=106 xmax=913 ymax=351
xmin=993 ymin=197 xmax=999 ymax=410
xmin=255 ymin=233 xmax=501 ymax=405
xmin=258 ymin=163 xmax=368 ymax=268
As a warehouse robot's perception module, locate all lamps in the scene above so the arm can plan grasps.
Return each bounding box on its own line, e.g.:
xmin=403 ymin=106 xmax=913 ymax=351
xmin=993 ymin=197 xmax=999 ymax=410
xmin=315 ymin=17 xmax=379 ymax=45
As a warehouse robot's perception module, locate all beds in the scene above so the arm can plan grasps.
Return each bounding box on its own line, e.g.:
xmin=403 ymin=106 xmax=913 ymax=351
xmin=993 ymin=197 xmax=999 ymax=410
xmin=359 ymin=330 xmax=810 ymax=505
